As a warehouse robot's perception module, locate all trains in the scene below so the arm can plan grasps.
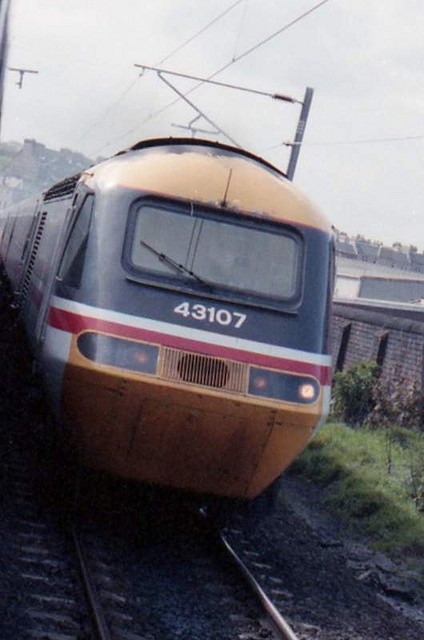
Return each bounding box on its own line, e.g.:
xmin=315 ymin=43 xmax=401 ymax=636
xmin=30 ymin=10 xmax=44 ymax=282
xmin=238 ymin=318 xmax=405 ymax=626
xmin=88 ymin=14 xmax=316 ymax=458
xmin=0 ymin=136 xmax=336 ymax=504
